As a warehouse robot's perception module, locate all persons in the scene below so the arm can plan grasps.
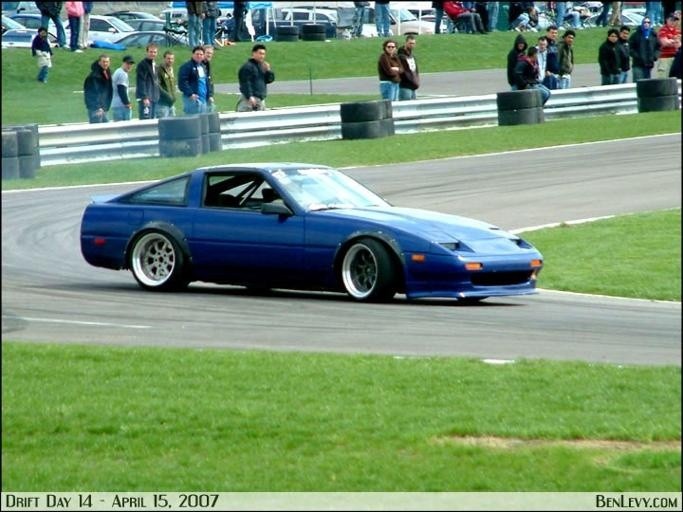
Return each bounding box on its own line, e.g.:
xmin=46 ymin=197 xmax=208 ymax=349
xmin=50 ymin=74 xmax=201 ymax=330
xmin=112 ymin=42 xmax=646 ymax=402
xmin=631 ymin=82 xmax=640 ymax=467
xmin=81 ymin=41 xmax=215 ymax=125
xmin=506 ymin=9 xmax=683 ymax=104
xmin=233 ymin=44 xmax=275 ymax=111
xmin=64 ymin=2 xmax=84 ymax=53
xmin=430 ymin=1 xmax=666 ymax=33
xmin=34 ymin=0 xmax=64 ymax=48
xmin=353 ymin=1 xmax=370 ymax=37
xmin=80 ymin=2 xmax=92 ymax=48
xmin=30 ymin=29 xmax=51 ymax=83
xmin=374 ymin=2 xmax=390 ymax=38
xmin=376 ymin=40 xmax=403 ymax=99
xmin=399 ymin=35 xmax=420 ymax=101
xmin=185 ymin=0 xmax=248 ymax=46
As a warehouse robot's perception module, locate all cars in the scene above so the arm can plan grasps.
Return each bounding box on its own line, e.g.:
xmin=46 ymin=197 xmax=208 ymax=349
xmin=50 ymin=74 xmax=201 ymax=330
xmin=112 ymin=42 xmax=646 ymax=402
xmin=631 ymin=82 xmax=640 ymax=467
xmin=112 ymin=31 xmax=204 ymax=47
xmin=50 ymin=15 xmax=139 ymax=43
xmin=104 ymin=10 xmax=160 ymax=24
xmin=32 ymin=13 xmax=55 ymax=28
xmin=2 ymin=28 xmax=57 ymax=45
xmin=216 ymin=2 xmax=662 ymax=43
xmin=1 ymin=14 xmax=27 ymax=33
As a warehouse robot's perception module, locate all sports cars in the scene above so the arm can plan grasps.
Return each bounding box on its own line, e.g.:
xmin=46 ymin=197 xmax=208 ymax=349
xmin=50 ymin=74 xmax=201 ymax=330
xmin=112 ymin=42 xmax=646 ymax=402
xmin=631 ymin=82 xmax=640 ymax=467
xmin=79 ymin=161 xmax=546 ymax=306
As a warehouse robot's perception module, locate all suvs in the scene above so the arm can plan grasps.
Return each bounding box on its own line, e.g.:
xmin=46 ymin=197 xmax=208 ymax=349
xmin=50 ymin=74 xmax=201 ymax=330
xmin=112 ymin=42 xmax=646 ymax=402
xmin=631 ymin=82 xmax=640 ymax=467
xmin=123 ymin=19 xmax=178 ymax=32
xmin=160 ymin=9 xmax=187 ymax=20
